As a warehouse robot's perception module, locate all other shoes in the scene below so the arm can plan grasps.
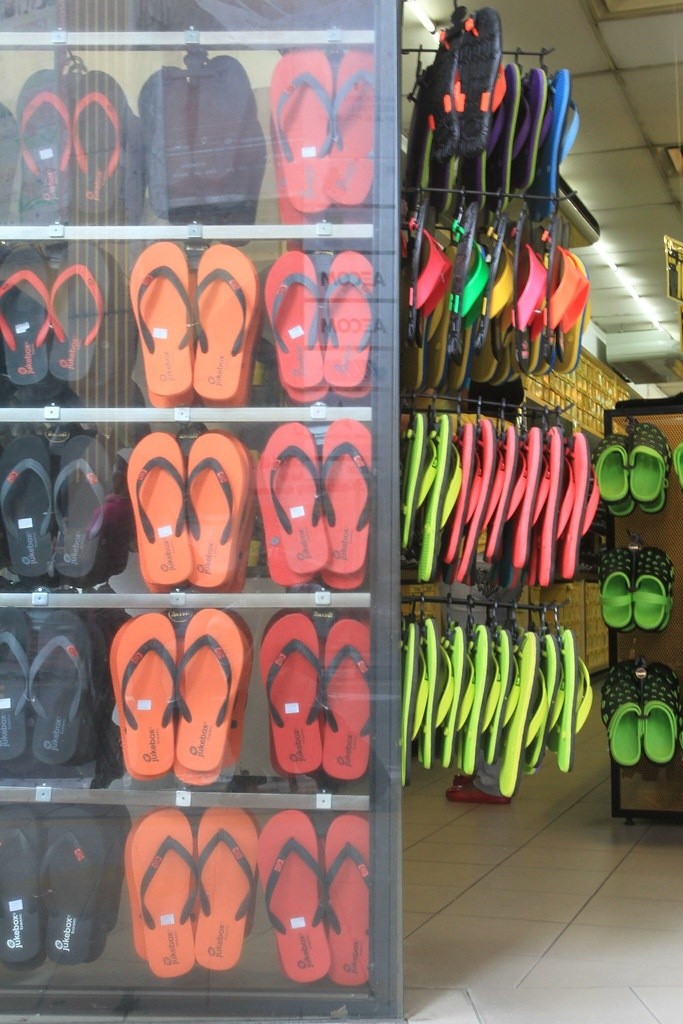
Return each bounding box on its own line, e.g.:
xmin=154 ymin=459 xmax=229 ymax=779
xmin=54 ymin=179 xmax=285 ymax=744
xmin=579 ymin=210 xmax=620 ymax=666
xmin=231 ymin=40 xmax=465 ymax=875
xmin=445 ymin=782 xmax=511 ymax=803
xmin=454 ymin=773 xmax=474 ymax=784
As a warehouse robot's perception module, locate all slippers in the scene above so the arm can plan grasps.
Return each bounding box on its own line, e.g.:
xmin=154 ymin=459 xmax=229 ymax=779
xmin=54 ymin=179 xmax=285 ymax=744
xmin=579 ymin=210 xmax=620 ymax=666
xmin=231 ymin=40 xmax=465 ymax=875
xmin=400 ymin=5 xmax=594 ymax=799
xmin=599 ymin=422 xmax=672 ymax=515
xmin=0 ymin=40 xmax=376 ymax=984
xmin=601 ymin=660 xmax=680 ymax=766
xmin=600 ymin=546 xmax=676 ymax=630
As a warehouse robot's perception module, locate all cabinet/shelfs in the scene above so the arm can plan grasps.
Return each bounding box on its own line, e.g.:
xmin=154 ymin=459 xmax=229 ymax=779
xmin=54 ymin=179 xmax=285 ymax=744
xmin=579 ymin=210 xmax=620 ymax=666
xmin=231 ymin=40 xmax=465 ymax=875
xmin=0 ymin=0 xmax=405 ymax=1024
